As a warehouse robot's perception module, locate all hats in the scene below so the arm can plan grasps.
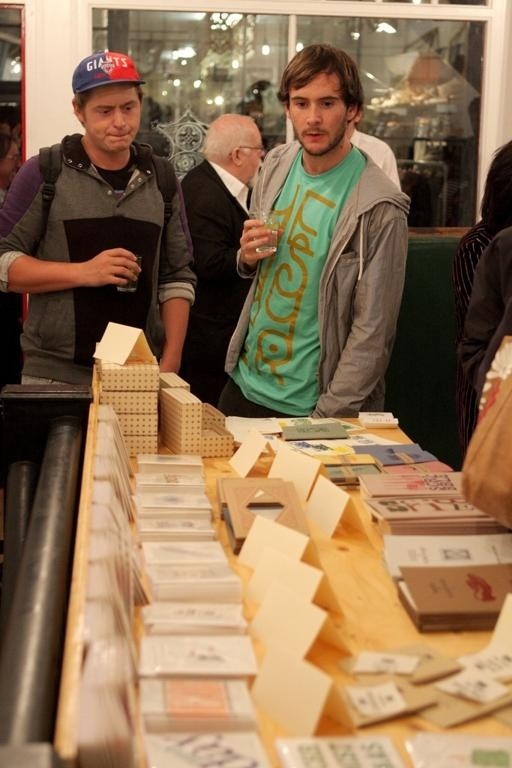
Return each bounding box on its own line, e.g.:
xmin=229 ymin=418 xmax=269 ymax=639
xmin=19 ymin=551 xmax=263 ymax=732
xmin=72 ymin=50 xmax=146 ymax=94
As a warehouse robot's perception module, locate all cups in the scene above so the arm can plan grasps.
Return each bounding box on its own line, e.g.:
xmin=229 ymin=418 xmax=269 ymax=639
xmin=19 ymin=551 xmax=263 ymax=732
xmin=115 ymin=251 xmax=142 ymax=293
xmin=250 ymin=209 xmax=277 ymax=257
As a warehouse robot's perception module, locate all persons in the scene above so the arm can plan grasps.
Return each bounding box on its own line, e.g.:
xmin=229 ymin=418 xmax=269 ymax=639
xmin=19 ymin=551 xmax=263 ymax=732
xmin=180 ymin=45 xmax=411 ymax=418
xmin=0 ymin=134 xmax=21 ymax=209
xmin=452 ymin=140 xmax=511 ymax=459
xmin=1 ymin=53 xmax=199 ymax=386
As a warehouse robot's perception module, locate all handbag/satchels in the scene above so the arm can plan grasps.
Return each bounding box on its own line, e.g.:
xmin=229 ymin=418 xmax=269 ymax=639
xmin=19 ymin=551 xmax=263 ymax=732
xmin=460 ymin=370 xmax=511 ymax=530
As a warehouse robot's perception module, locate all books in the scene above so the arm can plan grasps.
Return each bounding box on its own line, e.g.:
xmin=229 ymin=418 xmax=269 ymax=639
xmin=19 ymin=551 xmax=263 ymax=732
xmin=74 ymin=404 xmax=265 ymax=767
xmin=226 ymin=411 xmax=512 ymax=768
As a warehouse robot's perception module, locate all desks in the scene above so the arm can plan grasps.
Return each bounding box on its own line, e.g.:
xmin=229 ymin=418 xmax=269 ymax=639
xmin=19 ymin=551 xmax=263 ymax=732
xmin=49 ymin=358 xmax=512 ymax=767
